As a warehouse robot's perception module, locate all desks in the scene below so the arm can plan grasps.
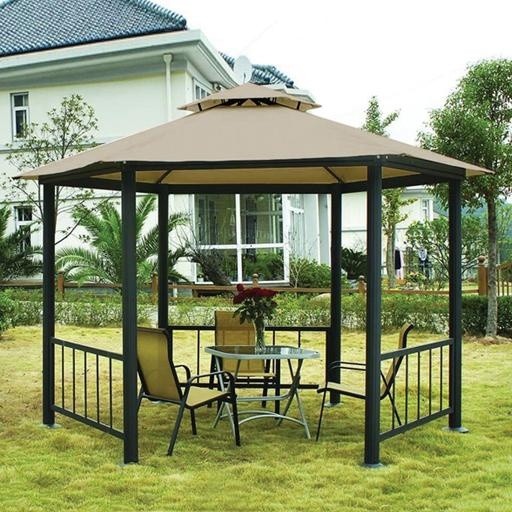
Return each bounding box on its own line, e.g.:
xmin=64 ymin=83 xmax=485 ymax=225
xmin=205 ymin=345 xmax=319 ymax=439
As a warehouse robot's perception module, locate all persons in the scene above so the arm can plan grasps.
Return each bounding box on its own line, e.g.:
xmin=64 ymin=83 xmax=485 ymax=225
xmin=416 ymin=246 xmax=431 ymax=278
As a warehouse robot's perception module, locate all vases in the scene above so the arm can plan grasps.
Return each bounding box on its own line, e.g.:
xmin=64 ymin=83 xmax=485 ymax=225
xmin=251 ymin=317 xmax=268 ymax=352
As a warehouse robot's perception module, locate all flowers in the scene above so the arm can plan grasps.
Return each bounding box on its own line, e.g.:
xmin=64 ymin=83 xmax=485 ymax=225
xmin=232 ymin=284 xmax=279 ymax=340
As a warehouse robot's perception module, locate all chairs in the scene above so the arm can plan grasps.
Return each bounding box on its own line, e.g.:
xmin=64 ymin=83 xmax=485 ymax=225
xmin=208 ymin=311 xmax=280 ymax=419
xmin=317 ymin=322 xmax=414 ymax=442
xmin=136 ymin=327 xmax=240 ymax=456
